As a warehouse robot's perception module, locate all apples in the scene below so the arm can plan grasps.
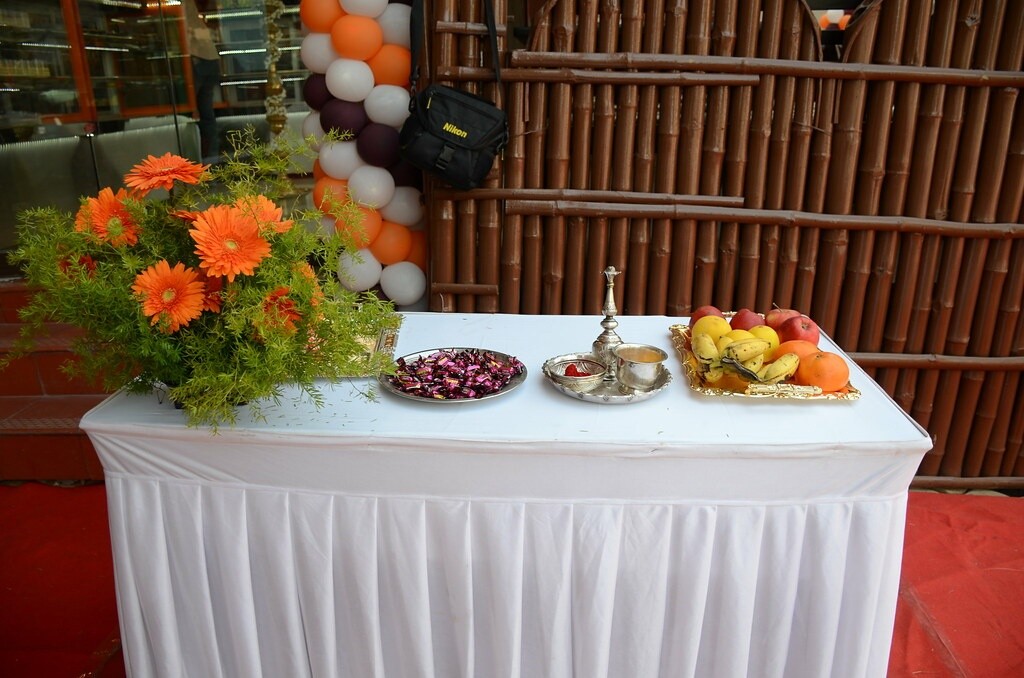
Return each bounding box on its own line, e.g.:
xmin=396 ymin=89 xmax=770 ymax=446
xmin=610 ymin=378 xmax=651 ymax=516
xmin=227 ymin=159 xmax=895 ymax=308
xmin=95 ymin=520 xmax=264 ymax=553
xmin=689 ymin=301 xmax=820 ymax=346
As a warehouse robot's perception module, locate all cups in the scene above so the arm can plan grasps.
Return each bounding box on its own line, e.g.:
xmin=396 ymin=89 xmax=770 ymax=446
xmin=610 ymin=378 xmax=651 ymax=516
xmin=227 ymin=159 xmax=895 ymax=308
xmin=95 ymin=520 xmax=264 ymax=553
xmin=612 ymin=342 xmax=668 ymax=395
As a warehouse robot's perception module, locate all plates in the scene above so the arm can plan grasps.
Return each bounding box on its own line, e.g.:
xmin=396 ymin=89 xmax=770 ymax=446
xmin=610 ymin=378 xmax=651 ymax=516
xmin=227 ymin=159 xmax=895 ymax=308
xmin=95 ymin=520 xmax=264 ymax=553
xmin=542 ymin=350 xmax=673 ymax=405
xmin=377 ymin=348 xmax=528 ymax=404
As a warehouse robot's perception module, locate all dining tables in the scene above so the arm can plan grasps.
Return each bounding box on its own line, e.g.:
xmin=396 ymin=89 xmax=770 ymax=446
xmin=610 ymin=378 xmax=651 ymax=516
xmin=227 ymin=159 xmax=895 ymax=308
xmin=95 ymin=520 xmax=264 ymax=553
xmin=79 ymin=310 xmax=933 ymax=678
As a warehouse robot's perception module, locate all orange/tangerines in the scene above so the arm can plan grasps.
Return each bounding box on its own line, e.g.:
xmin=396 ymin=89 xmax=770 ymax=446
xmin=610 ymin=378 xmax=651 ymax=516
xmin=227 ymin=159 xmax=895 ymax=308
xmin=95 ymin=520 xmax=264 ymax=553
xmin=777 ymin=340 xmax=848 ymax=391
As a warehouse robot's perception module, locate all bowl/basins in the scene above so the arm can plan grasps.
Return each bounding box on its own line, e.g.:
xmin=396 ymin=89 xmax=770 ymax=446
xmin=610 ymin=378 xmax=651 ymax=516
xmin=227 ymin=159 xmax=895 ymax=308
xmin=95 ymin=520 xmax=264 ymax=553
xmin=547 ymin=355 xmax=609 ymax=393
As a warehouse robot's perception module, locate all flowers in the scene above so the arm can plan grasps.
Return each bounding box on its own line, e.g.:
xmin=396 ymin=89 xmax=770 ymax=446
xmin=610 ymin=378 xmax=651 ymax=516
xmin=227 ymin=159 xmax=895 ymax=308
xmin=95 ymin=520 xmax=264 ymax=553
xmin=7 ymin=129 xmax=402 ymax=432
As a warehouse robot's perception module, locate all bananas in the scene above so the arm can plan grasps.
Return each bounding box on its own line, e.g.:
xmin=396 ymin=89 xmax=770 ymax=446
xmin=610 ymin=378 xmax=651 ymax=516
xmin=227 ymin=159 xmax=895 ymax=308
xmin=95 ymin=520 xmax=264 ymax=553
xmin=692 ymin=333 xmax=798 ymax=383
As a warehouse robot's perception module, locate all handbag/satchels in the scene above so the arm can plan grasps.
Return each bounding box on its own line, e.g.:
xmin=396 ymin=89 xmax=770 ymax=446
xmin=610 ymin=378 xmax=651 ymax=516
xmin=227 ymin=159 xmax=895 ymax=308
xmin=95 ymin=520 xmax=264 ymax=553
xmin=399 ymin=84 xmax=509 ymax=190
